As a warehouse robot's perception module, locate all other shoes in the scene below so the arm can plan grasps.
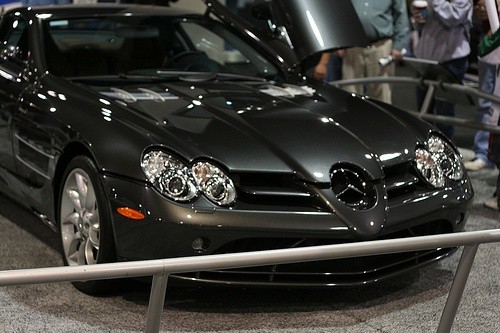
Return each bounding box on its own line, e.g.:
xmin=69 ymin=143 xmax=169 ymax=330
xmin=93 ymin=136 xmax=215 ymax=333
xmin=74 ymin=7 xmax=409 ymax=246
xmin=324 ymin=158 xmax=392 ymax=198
xmin=464 ymin=157 xmax=495 ymax=172
xmin=483 ymin=197 xmax=498 ymax=211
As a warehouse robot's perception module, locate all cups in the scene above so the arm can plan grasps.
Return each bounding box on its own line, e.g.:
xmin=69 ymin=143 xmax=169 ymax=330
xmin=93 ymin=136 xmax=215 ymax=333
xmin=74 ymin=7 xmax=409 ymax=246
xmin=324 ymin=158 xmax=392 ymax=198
xmin=412 ymin=0 xmax=428 ymax=22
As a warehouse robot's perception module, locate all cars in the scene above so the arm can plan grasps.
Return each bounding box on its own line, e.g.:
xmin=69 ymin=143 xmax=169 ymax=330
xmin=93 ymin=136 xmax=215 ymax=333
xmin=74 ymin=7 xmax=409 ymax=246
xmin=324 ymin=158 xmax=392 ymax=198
xmin=0 ymin=0 xmax=475 ymax=296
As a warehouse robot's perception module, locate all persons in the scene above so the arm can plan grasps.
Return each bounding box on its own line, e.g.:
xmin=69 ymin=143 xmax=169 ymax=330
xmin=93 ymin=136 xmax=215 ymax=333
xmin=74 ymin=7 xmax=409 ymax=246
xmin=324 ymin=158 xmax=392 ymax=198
xmin=314 ymin=0 xmax=500 ymax=210
xmin=339 ymin=0 xmax=410 ymax=104
